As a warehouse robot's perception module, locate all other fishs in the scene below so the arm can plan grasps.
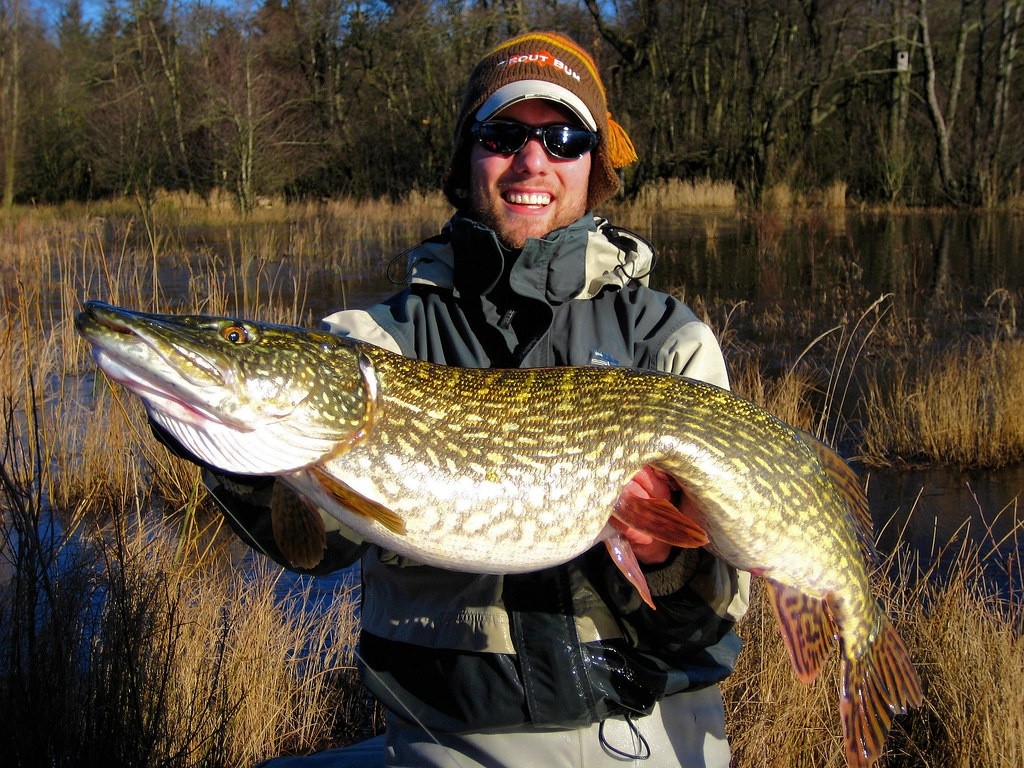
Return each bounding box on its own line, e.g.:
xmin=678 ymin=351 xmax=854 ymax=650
xmin=75 ymin=300 xmax=924 ymax=768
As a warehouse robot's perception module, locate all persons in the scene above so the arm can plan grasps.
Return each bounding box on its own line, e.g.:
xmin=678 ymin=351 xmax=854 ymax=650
xmin=200 ymin=34 xmax=751 ymax=768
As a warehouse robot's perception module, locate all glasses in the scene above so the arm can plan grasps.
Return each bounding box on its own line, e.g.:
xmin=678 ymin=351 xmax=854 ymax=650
xmin=470 ymin=117 xmax=601 ymax=159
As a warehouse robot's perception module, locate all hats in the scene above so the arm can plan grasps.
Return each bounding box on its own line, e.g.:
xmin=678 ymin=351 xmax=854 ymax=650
xmin=440 ymin=32 xmax=637 ymax=215
